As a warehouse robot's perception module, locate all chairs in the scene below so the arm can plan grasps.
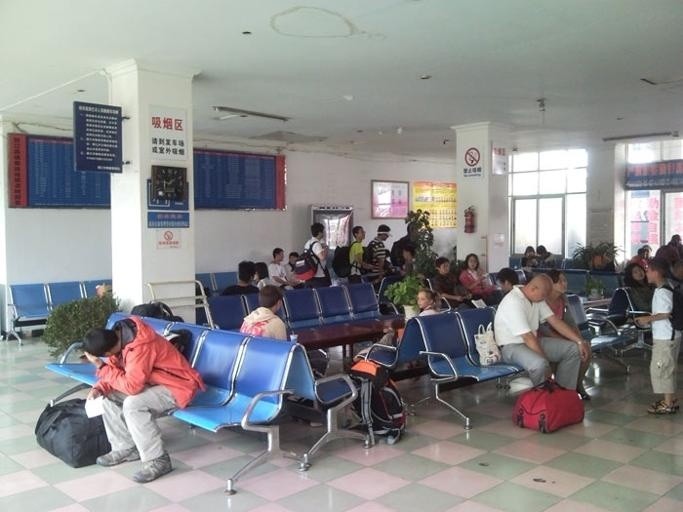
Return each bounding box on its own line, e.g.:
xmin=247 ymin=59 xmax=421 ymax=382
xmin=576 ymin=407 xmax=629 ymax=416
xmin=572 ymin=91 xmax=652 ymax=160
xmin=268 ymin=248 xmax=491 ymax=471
xmin=508 ymin=254 xmax=627 ymax=298
xmin=7 ymin=272 xmax=239 ymax=347
xmin=196 ymin=269 xmax=527 ymax=357
xmin=357 ymin=286 xmax=651 ymax=436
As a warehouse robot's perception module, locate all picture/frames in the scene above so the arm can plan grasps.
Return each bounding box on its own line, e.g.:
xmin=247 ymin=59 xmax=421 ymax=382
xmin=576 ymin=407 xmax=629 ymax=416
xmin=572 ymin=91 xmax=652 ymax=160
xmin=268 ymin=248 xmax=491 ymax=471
xmin=368 ymin=177 xmax=412 ymax=222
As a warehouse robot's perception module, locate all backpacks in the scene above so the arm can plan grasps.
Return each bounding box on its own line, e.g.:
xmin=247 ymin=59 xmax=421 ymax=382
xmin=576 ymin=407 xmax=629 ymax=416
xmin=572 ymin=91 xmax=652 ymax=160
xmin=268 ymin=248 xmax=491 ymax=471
xmin=349 ymin=359 xmax=406 ymax=445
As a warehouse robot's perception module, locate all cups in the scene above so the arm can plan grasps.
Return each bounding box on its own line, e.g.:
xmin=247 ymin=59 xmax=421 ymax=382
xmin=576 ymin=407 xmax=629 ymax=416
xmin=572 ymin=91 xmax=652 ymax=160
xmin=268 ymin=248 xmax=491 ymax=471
xmin=289 ymin=334 xmax=298 ymax=343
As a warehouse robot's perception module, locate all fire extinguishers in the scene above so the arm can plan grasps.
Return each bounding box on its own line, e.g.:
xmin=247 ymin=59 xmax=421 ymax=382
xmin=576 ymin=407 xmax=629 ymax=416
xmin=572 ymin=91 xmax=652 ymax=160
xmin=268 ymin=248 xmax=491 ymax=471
xmin=464 ymin=206 xmax=475 ymax=233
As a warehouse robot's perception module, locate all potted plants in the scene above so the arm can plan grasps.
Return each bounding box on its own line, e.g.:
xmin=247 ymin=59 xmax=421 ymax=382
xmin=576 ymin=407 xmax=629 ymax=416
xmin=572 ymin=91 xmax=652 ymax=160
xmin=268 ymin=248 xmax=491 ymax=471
xmin=569 ymin=239 xmax=626 ymax=272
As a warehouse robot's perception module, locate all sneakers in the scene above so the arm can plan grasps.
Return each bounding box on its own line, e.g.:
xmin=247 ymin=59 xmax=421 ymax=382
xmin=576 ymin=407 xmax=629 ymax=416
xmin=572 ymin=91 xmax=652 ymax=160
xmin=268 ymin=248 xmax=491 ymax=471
xmin=134 ymin=452 xmax=172 ymax=483
xmin=648 ymin=399 xmax=679 ymax=414
xmin=96 ymin=448 xmax=139 ymax=466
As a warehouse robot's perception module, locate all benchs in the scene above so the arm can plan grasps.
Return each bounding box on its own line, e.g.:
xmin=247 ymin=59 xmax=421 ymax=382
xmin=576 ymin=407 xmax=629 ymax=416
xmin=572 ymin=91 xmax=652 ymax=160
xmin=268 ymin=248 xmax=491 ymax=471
xmin=43 ymin=313 xmax=371 ymax=498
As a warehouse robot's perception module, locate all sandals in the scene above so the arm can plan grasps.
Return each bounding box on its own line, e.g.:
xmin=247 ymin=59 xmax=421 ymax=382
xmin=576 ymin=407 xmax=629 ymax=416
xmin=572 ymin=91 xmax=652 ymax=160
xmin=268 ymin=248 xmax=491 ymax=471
xmin=577 ymin=384 xmax=590 ymax=400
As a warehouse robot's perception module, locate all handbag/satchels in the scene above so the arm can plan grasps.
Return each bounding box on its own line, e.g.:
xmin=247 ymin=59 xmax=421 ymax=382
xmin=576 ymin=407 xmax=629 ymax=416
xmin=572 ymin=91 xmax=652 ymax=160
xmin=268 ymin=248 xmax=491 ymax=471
xmin=333 ymin=241 xmax=363 ymax=277
xmin=474 ymin=322 xmax=501 ymax=366
xmin=132 ymin=302 xmax=183 ymax=322
xmin=324 ymin=266 xmax=332 ymax=285
xmin=36 ymin=398 xmax=111 ymax=468
xmin=513 ymin=379 xmax=585 ymax=433
xmin=295 ymin=241 xmax=317 ymax=280
xmin=361 ymin=245 xmax=374 ymax=274
xmin=156 ymin=330 xmax=191 ymax=357
xmin=660 ymin=285 xmax=683 ymax=340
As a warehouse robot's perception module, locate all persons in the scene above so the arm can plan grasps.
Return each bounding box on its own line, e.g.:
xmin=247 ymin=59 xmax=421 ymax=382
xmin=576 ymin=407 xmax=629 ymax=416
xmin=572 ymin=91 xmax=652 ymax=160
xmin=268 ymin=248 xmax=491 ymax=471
xmin=552 ymin=236 xmax=682 ymax=414
xmin=381 ymin=252 xmax=520 ymax=345
xmin=78 ymin=315 xmax=206 ymax=487
xmin=522 ymin=245 xmax=556 ymax=279
xmin=218 ymin=219 xmax=422 ymax=296
xmin=240 ymin=285 xmax=288 ymax=342
xmin=494 ymin=275 xmax=590 ymax=390
